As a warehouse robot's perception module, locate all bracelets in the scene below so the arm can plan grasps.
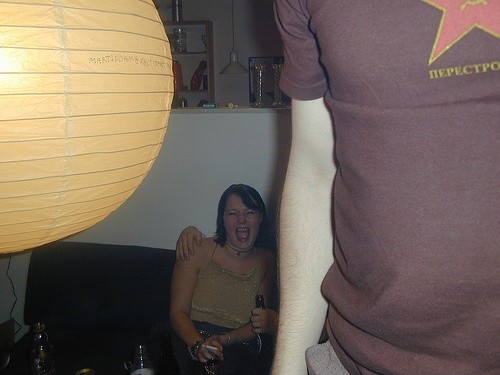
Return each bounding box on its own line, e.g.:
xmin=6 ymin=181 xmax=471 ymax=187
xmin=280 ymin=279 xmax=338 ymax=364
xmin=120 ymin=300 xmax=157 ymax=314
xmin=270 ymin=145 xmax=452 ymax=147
xmin=227 ymin=331 xmax=231 ymax=345
xmin=191 ymin=340 xmax=205 ymax=356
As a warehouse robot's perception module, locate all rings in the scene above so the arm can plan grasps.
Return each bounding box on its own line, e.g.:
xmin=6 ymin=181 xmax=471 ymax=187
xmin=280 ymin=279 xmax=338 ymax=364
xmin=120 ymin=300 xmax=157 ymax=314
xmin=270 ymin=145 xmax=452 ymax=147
xmin=226 ymin=241 xmax=254 ymax=255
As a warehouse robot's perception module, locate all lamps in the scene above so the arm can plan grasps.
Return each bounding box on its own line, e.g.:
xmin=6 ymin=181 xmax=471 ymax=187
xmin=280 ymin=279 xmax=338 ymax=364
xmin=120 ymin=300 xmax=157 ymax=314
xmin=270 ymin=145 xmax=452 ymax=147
xmin=219 ymin=0 xmax=249 ymax=75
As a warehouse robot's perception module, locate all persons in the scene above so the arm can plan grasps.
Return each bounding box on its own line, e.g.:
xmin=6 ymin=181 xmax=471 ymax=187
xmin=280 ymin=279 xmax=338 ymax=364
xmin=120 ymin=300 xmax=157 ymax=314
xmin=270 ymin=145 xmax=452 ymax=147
xmin=176 ymin=226 xmax=329 ymax=344
xmin=169 ymin=184 xmax=276 ymax=375
xmin=271 ymin=1 xmax=500 ymax=375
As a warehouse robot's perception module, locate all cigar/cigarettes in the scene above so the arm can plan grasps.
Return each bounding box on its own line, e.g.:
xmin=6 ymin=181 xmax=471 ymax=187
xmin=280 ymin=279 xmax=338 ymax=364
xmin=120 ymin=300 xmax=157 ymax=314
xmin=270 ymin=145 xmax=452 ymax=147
xmin=202 ymin=345 xmax=223 ymax=352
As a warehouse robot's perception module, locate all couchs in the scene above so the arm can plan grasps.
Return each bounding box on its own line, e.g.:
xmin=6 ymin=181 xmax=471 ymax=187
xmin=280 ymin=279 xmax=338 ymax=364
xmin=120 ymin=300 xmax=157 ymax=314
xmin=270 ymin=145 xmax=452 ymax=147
xmin=0 ymin=242 xmax=178 ymax=375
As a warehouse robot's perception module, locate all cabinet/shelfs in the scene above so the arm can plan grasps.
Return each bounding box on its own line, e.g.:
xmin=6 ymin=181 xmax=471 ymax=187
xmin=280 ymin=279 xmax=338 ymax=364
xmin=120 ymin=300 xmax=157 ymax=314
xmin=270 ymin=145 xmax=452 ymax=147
xmin=163 ymin=20 xmax=215 ymax=103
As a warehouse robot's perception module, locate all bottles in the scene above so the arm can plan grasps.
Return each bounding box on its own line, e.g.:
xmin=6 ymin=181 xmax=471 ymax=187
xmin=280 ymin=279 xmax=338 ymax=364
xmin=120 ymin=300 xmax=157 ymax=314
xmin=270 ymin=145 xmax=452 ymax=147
xmin=256 ymin=295 xmax=271 ymax=359
xmin=131 ymin=344 xmax=154 ymax=375
xmin=155 ymin=330 xmax=181 ymax=375
xmin=30 ymin=322 xmax=56 ymax=375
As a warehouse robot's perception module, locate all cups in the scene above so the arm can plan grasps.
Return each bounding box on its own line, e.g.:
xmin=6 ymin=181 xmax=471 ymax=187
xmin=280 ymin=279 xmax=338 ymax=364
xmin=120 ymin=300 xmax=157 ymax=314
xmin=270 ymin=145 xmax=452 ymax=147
xmin=172 ymin=60 xmax=182 ymax=90
xmin=172 ymin=0 xmax=182 ymax=22
xmin=174 ymin=28 xmax=187 ymax=55
